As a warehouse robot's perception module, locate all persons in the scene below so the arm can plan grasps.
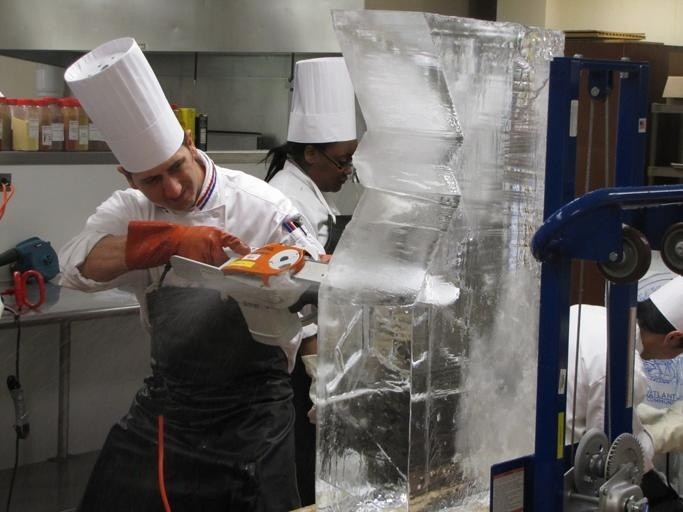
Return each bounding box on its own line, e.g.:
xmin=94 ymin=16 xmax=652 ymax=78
xmin=258 ymin=140 xmax=358 ymax=507
xmin=57 ymin=129 xmax=326 ymax=512
xmin=565 ymin=299 xmax=683 ymax=512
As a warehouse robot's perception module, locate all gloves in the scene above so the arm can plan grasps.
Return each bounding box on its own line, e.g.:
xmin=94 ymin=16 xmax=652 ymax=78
xmin=124 ymin=220 xmax=251 ymax=270
xmin=637 ymin=400 xmax=668 ymax=424
xmin=301 ymin=354 xmax=318 ymax=424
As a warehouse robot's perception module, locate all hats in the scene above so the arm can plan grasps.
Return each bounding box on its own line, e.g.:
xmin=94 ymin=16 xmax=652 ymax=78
xmin=648 ymin=276 xmax=683 ymax=334
xmin=287 ymin=57 xmax=357 ymax=143
xmin=64 ymin=37 xmax=184 ymax=173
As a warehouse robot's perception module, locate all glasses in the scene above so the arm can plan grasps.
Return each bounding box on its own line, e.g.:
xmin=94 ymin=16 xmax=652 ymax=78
xmin=310 ymin=145 xmax=353 ymax=173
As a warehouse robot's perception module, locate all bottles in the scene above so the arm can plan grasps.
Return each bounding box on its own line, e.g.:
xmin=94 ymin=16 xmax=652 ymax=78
xmin=0 ymin=97 xmax=112 ymax=152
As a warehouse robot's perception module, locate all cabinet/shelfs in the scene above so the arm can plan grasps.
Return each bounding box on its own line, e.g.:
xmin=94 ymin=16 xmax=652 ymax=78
xmin=0 ymin=0 xmax=375 ymax=511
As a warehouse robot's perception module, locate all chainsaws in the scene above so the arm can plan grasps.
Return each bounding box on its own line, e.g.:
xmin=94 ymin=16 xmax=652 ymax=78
xmin=170 ymin=240 xmax=461 ymax=313
